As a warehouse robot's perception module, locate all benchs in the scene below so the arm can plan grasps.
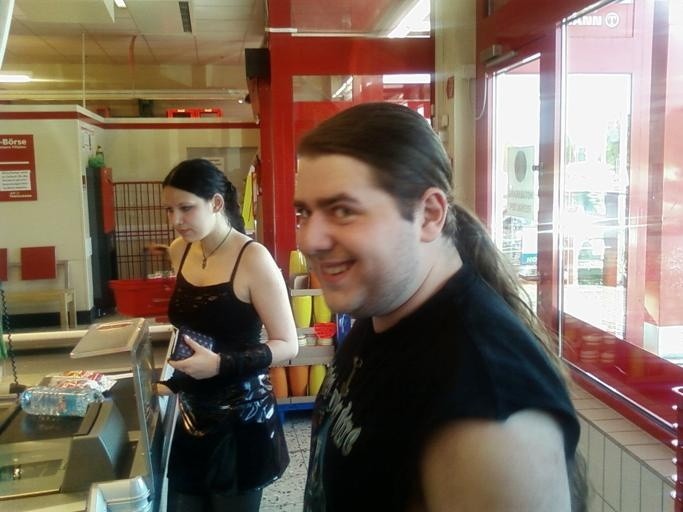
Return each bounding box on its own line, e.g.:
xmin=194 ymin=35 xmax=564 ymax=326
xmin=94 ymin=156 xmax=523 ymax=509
xmin=0 ymin=246 xmax=78 ymax=331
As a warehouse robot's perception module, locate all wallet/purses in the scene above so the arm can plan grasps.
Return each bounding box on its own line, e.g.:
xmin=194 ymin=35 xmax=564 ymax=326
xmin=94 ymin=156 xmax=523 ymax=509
xmin=173 ymin=327 xmax=216 ymax=361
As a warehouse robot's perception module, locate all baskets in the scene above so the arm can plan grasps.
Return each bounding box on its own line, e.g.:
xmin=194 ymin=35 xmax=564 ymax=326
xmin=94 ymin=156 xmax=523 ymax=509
xmin=109 ymin=277 xmax=176 ymax=317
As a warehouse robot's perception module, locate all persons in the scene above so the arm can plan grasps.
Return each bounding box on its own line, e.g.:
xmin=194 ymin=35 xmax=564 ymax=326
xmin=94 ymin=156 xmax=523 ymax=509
xmin=291 ymin=102 xmax=591 ymax=512
xmin=144 ymin=156 xmax=299 ymax=512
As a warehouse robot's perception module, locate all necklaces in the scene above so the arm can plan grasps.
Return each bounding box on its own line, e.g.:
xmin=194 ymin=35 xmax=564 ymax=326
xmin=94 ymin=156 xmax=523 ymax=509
xmin=200 ymin=227 xmax=233 ymax=270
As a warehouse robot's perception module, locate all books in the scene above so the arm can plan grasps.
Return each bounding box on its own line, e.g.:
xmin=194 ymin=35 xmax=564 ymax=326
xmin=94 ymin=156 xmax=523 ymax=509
xmin=70 ymin=318 xmax=145 ymax=358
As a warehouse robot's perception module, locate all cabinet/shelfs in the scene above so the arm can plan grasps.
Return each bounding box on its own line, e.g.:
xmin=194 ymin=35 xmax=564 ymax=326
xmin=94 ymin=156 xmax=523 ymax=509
xmin=260 ymin=287 xmax=338 ymax=424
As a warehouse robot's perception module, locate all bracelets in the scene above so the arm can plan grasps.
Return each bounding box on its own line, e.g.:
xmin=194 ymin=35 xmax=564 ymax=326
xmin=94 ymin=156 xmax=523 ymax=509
xmin=216 ymin=342 xmax=272 ymax=374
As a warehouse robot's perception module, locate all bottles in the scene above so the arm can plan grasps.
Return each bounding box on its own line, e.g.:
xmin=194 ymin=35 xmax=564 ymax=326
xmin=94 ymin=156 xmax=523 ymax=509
xmin=16 ymin=384 xmax=105 ymax=418
